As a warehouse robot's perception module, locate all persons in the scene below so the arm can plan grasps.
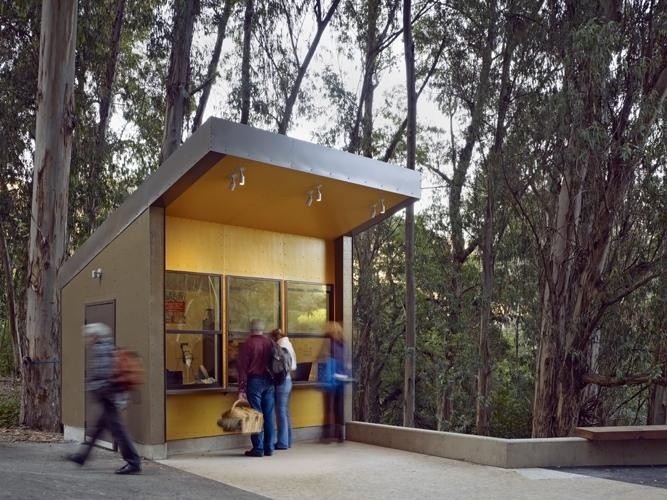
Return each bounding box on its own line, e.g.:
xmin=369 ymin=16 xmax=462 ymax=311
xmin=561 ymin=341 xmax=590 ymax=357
xmin=59 ymin=321 xmax=143 ymax=474
xmin=240 ymin=317 xmax=296 ymax=457
xmin=312 ymin=319 xmax=344 ymax=444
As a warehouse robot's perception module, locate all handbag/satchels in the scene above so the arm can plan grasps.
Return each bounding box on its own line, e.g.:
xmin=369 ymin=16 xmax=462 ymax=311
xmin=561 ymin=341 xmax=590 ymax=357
xmin=314 ymin=358 xmax=338 ymax=391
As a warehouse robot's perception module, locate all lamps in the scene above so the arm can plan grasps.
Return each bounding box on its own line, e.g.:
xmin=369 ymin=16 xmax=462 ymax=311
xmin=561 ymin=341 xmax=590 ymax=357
xmin=368 ymin=198 xmax=386 ymax=220
xmin=304 ymin=184 xmax=322 ymax=208
xmin=227 ymin=165 xmax=247 ymax=192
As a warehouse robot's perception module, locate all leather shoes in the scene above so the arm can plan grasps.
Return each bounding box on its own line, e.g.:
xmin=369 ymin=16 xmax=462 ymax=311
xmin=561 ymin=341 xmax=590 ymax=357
xmin=67 ymin=453 xmax=84 ymax=465
xmin=244 ymin=450 xmax=273 ymax=457
xmin=115 ymin=463 xmax=141 ymax=474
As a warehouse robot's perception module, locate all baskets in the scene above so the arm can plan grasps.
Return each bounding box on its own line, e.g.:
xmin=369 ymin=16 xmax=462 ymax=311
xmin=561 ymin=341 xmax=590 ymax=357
xmin=220 ymin=399 xmax=264 ymax=434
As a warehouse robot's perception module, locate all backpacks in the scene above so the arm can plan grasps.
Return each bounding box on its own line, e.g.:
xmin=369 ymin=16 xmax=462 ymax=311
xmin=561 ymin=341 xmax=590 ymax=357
xmin=110 ymin=347 xmax=146 ymax=393
xmin=266 ymin=338 xmax=292 ymax=385
